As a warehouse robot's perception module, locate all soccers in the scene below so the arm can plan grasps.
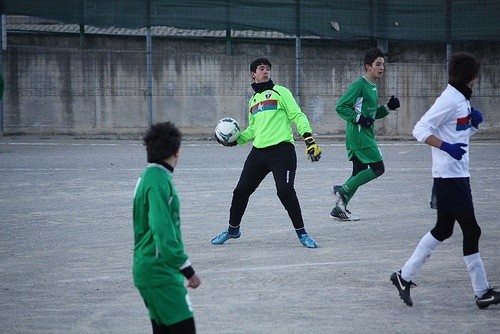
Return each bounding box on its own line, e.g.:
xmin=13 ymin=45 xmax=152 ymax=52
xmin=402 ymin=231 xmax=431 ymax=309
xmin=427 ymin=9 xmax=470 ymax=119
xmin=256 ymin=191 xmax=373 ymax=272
xmin=215 ymin=118 xmax=241 ymax=143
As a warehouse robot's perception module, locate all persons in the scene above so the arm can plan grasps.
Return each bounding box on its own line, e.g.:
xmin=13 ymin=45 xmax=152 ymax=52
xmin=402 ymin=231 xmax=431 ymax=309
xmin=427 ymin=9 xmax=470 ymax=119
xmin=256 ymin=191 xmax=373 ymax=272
xmin=390 ymin=51 xmax=500 ymax=309
xmin=132 ymin=122 xmax=201 ymax=334
xmin=211 ymin=58 xmax=321 ymax=248
xmin=330 ymin=47 xmax=400 ymax=220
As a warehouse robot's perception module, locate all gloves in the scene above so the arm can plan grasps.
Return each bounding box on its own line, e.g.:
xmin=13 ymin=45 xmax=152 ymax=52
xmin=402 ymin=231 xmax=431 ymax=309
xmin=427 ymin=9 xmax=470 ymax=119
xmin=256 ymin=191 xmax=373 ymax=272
xmin=386 ymin=94 xmax=401 ymax=110
xmin=357 ymin=114 xmax=375 ymax=129
xmin=302 ymin=132 xmax=322 ymax=162
xmin=468 ymin=107 xmax=483 ymax=130
xmin=439 ymin=141 xmax=468 ymax=161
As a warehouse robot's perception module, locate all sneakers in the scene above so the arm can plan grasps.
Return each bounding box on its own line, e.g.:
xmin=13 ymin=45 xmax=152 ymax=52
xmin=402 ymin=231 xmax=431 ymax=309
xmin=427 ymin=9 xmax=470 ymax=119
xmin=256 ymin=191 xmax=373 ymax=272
xmin=210 ymin=228 xmax=241 ymax=245
xmin=330 ymin=185 xmax=348 ymax=207
xmin=328 ymin=205 xmax=360 ymax=222
xmin=389 ymin=269 xmax=418 ymax=307
xmin=294 ymin=233 xmax=316 ymax=248
xmin=474 ymin=287 xmax=500 ymax=309
